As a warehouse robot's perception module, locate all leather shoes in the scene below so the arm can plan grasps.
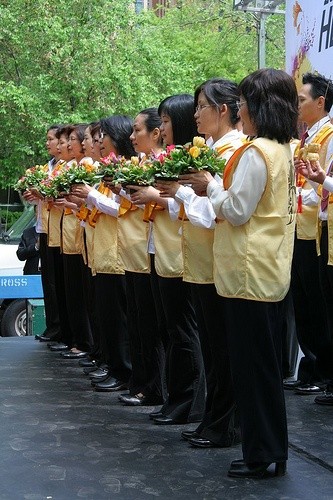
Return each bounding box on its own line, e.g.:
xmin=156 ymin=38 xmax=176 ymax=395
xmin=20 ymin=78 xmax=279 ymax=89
xmin=181 ymin=430 xmax=216 ymax=447
xmin=47 ymin=342 xmax=69 ymax=350
xmin=118 ymin=392 xmax=148 ymax=406
xmin=294 ymin=383 xmax=326 ymax=394
xmin=60 ymin=348 xmax=88 ymax=358
xmin=91 ymin=374 xmax=131 ymax=391
xmin=83 ymin=364 xmax=108 ymax=377
xmin=78 ymin=357 xmax=94 ymax=367
xmin=282 ymin=378 xmax=304 ymax=389
xmin=314 ymin=395 xmax=333 ymax=406
xmin=149 ymin=412 xmax=178 ymax=426
xmin=35 ymin=334 xmax=53 ymax=341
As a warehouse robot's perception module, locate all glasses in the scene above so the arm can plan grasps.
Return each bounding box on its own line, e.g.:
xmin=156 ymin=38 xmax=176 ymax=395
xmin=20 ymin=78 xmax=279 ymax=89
xmin=99 ymin=132 xmax=108 ymax=139
xmin=67 ymin=137 xmax=79 ymax=142
xmin=236 ymin=100 xmax=246 ymax=110
xmin=196 ymin=104 xmax=215 ymax=112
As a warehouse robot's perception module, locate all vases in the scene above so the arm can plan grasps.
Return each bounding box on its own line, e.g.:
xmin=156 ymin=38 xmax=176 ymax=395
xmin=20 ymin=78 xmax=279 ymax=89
xmin=58 ymin=182 xmax=74 ymax=193
xmin=122 ymin=181 xmax=150 ymax=195
xmin=105 ymin=172 xmax=113 ymax=182
xmin=155 ymin=173 xmax=179 ymax=182
xmin=20 ymin=189 xmax=27 ymax=196
xmin=51 ymin=195 xmax=64 ymax=201
xmin=75 ymin=179 xmax=93 ymax=186
xmin=184 ymin=166 xmax=215 ymax=178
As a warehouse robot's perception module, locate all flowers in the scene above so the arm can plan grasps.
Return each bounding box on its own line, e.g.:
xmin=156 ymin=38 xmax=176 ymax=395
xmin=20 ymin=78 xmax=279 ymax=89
xmin=13 ymin=136 xmax=226 ymax=199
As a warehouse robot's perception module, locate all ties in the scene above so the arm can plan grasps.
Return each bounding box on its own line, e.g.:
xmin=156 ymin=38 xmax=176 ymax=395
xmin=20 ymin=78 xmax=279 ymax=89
xmin=300 ymin=132 xmax=309 ymax=148
xmin=321 ymin=161 xmax=333 ymax=212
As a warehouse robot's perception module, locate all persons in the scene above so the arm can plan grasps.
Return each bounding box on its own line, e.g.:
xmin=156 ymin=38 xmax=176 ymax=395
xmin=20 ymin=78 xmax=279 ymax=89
xmin=22 ymin=68 xmax=298 ymax=478
xmin=281 ymin=70 xmax=333 ymax=406
xmin=17 ymin=205 xmax=42 ymax=275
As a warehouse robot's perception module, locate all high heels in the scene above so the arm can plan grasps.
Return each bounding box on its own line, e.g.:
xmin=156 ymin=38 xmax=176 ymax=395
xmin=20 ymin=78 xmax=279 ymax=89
xmin=227 ymin=460 xmax=287 ymax=478
